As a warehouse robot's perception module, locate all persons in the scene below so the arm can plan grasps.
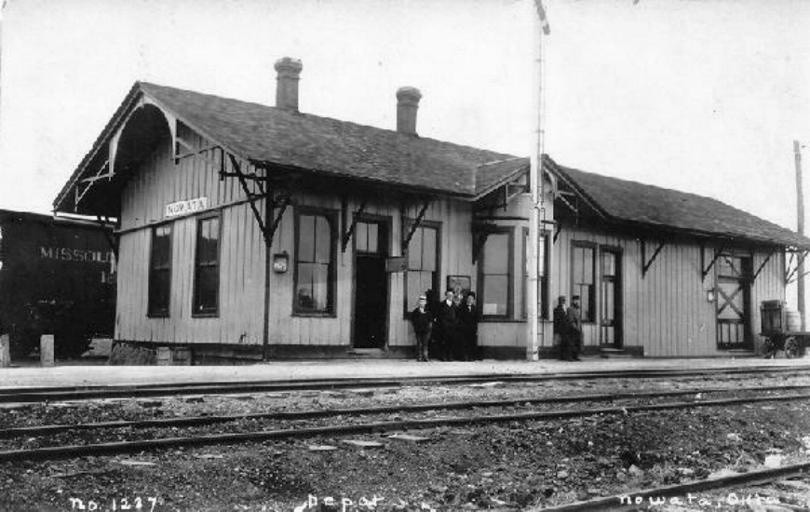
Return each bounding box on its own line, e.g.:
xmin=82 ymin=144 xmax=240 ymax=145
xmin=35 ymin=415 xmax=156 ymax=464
xmin=566 ymin=295 xmax=581 ymax=363
xmin=412 ymin=292 xmax=437 ymax=363
xmin=435 ymin=287 xmax=458 ymax=360
xmin=553 ymin=294 xmax=581 ymax=362
xmin=458 ymin=292 xmax=479 ymax=361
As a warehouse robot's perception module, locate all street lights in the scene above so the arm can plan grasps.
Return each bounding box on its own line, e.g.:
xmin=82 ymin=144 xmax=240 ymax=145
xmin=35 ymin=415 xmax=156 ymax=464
xmin=526 ymin=0 xmax=551 ymax=363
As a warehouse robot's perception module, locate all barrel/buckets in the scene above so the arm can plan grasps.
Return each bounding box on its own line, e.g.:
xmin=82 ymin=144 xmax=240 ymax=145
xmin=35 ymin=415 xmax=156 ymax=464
xmin=786 ymin=311 xmax=801 ymax=331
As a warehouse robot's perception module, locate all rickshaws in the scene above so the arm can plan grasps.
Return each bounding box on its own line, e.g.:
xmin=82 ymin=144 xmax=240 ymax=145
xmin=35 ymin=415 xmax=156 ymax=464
xmin=760 ymin=330 xmax=810 ymax=360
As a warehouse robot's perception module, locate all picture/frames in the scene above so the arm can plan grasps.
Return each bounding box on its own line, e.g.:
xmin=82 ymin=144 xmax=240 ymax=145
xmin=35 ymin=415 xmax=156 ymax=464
xmin=446 ymin=274 xmax=472 ymax=295
